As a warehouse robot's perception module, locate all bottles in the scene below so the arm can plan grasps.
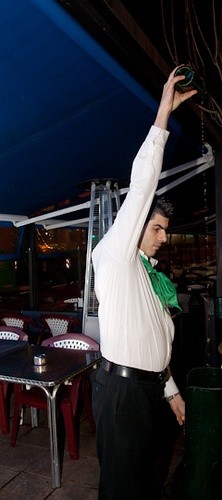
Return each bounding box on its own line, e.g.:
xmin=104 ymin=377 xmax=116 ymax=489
xmin=168 ymin=63 xmax=198 ymax=91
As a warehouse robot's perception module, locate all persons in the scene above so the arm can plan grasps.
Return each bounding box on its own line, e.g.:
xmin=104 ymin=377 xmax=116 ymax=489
xmin=91 ymin=64 xmax=198 ymax=496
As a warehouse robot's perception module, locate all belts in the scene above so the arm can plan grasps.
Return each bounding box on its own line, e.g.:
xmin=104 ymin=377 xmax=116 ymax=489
xmin=101 ymin=358 xmax=170 ymax=382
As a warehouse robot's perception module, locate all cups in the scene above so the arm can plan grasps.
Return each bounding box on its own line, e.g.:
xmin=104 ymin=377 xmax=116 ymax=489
xmin=34 ymin=354 xmax=46 ymax=374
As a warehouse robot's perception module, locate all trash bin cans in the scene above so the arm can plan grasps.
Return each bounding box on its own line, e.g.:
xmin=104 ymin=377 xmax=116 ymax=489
xmin=185 ymin=367 xmax=222 ymax=500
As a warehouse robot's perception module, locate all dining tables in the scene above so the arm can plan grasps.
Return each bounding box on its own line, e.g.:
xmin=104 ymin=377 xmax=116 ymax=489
xmin=18 ymin=302 xmax=83 ymax=345
xmin=0 ymin=339 xmax=103 ymax=488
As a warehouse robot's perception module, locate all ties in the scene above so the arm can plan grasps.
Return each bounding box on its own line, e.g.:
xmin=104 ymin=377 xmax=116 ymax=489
xmin=140 ymin=255 xmax=182 ymax=313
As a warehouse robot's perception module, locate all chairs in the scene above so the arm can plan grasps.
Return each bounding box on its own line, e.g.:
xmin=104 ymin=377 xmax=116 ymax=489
xmin=0 ymin=257 xmax=217 ymax=460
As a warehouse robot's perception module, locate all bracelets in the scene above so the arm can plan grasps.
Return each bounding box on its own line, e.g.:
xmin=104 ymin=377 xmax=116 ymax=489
xmin=165 ymin=391 xmax=180 ymax=401
